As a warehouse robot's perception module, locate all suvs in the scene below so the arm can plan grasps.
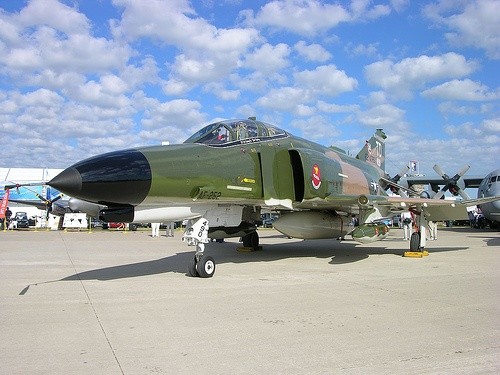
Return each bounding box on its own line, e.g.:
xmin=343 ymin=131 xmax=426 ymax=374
xmin=14 ymin=211 xmax=30 ymax=229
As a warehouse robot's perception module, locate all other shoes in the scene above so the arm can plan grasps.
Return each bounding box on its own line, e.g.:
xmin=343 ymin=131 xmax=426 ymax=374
xmin=403 ymin=237 xmax=411 ymax=240
xmin=429 ymin=237 xmax=438 ymax=241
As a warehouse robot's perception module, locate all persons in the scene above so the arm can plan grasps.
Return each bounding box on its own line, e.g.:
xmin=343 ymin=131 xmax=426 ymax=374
xmin=151 ymin=222 xmax=160 ymax=238
xmin=166 ymin=221 xmax=174 ymax=237
xmin=469 ymin=211 xmax=485 ymax=229
xmin=5 ymin=207 xmax=12 ymax=221
xmin=401 ymin=213 xmax=412 ymax=240
xmin=428 ymin=221 xmax=438 ymax=240
xmin=117 ymin=222 xmax=125 ymax=230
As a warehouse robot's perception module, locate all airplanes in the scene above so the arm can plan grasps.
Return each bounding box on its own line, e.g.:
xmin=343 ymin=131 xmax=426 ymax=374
xmin=380 ymin=163 xmax=500 ymax=231
xmin=6 ymin=116 xmax=500 ymax=278
